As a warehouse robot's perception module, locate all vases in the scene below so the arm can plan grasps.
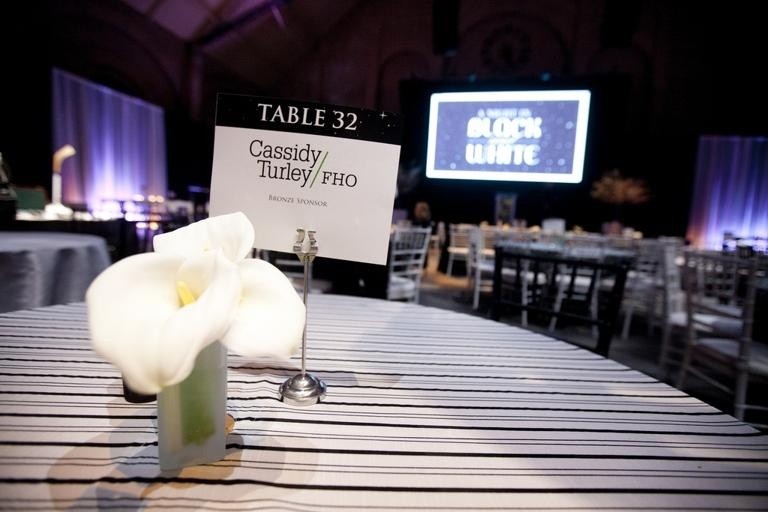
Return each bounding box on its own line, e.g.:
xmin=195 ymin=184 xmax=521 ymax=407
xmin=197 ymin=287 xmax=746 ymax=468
xmin=157 ymin=339 xmax=228 ymax=472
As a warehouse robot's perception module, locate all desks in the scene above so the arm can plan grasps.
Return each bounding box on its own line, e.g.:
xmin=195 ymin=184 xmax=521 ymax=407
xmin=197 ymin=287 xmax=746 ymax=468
xmin=0 ymin=231 xmax=111 ymax=315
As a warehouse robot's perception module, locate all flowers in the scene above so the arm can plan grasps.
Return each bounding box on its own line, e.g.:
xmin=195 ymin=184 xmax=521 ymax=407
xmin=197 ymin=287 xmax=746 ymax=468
xmin=84 ymin=209 xmax=306 ymax=446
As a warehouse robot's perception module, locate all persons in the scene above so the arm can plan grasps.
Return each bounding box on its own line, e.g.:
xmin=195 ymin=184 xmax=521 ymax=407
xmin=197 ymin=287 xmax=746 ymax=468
xmin=412 ymin=202 xmax=432 ymax=229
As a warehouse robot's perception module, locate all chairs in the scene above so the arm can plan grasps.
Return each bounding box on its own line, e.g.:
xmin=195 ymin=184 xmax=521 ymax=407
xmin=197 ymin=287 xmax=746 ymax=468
xmin=389 ymin=220 xmax=637 ymax=358
xmin=624 ymin=230 xmax=766 ymax=429
